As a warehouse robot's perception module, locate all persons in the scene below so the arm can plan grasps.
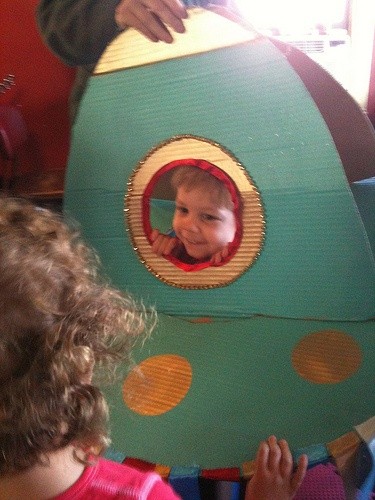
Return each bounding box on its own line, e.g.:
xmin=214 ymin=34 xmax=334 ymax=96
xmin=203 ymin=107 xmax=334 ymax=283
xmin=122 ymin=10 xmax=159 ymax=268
xmin=36 ymin=0 xmax=246 ymax=132
xmin=150 ymin=166 xmax=238 ymax=267
xmin=0 ymin=195 xmax=309 ymax=500
